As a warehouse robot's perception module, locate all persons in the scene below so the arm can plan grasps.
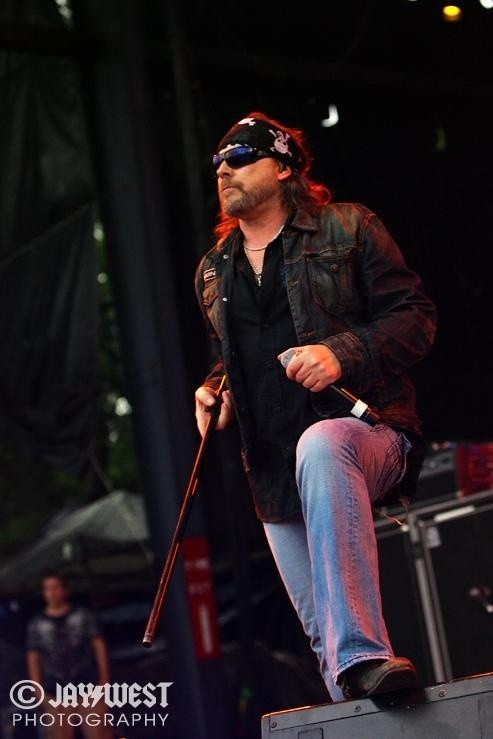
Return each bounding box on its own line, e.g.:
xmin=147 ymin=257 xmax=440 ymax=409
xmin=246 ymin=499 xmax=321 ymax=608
xmin=183 ymin=108 xmax=438 ymax=707
xmin=25 ymin=572 xmax=115 ymax=738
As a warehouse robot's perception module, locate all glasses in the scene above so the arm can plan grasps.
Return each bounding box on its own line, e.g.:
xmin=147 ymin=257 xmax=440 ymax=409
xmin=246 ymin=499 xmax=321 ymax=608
xmin=210 ymin=144 xmax=274 ymax=170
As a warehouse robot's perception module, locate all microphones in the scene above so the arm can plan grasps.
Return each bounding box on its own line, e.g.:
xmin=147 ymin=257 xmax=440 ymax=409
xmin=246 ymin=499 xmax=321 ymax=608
xmin=279 ymin=347 xmax=382 ymax=428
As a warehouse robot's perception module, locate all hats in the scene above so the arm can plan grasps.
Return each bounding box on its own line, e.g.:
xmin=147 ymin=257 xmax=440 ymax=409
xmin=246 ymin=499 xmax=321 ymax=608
xmin=215 ymin=112 xmax=307 ymax=169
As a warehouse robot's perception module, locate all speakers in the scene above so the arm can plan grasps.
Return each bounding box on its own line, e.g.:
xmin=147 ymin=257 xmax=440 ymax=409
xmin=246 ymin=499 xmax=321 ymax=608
xmin=372 ymin=486 xmax=493 ymax=684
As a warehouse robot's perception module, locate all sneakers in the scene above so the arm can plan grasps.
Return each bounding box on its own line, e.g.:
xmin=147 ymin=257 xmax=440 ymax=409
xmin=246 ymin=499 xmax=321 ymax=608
xmin=339 ymin=652 xmax=420 ymax=704
